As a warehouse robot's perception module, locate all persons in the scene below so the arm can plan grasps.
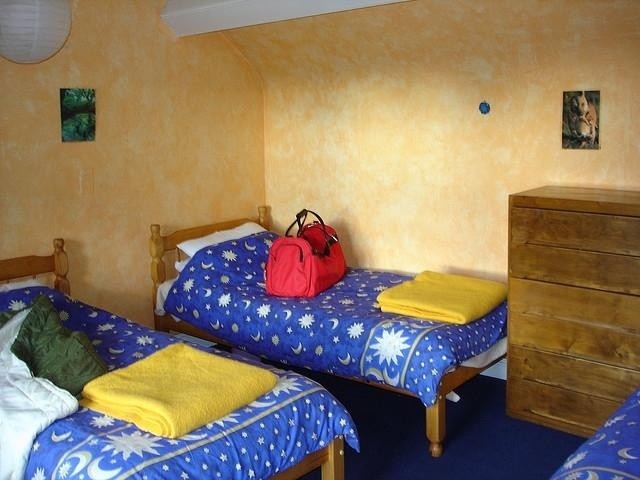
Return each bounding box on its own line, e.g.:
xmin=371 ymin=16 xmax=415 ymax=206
xmin=569 ymin=91 xmax=597 ymax=146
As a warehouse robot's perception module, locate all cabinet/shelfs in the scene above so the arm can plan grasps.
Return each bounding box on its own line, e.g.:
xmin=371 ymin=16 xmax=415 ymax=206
xmin=504 ymin=185 xmax=640 ymax=438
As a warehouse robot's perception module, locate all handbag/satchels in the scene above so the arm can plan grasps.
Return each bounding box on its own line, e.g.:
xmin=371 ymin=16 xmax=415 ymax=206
xmin=263 ymin=209 xmax=347 ymax=297
